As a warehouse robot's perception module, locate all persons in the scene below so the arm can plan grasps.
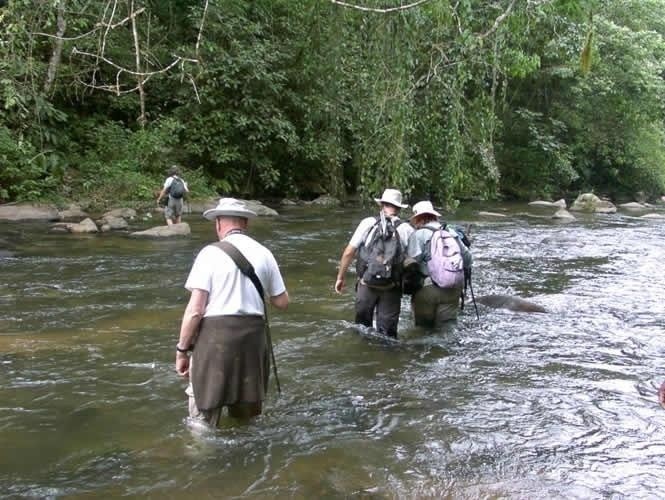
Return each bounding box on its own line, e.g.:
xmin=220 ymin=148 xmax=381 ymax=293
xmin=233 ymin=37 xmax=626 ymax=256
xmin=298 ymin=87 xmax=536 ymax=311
xmin=157 ymin=164 xmax=190 ymax=225
xmin=336 ymin=190 xmax=416 ymax=341
xmin=404 ymin=198 xmax=473 ymax=329
xmin=175 ymin=197 xmax=289 ymax=429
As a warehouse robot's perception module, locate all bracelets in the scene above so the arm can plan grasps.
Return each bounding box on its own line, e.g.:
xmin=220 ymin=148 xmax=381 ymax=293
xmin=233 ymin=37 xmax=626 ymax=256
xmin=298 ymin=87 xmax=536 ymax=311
xmin=175 ymin=344 xmax=190 ymax=353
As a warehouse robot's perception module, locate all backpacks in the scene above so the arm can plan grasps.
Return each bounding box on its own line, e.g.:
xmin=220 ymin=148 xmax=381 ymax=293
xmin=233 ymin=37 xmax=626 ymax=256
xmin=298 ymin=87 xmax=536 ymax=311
xmin=170 ymin=176 xmax=183 ymax=198
xmin=356 ymin=214 xmax=410 ymax=290
xmin=417 ymin=223 xmax=465 ymax=289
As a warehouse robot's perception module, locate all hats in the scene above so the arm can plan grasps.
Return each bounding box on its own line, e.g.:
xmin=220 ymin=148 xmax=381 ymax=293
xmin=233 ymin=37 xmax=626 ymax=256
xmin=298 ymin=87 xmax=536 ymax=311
xmin=409 ymin=200 xmax=442 ymax=219
xmin=203 ymin=197 xmax=257 ymax=221
xmin=374 ymin=188 xmax=409 ymax=209
